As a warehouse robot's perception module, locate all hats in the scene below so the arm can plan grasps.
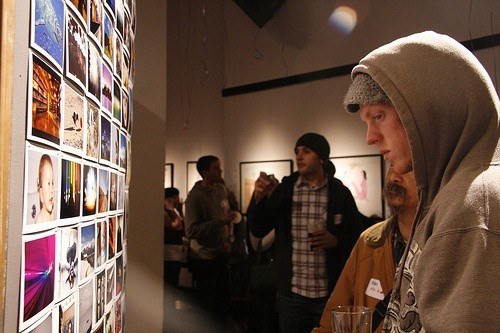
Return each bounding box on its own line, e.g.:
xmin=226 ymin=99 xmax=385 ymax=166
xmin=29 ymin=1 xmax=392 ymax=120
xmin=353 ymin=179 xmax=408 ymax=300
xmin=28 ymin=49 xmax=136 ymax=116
xmin=324 ymin=160 xmax=335 ymax=176
xmin=343 ymin=73 xmax=392 ymax=113
xmin=294 ymin=133 xmax=330 ymax=164
xmin=165 ymin=187 xmax=179 ymax=198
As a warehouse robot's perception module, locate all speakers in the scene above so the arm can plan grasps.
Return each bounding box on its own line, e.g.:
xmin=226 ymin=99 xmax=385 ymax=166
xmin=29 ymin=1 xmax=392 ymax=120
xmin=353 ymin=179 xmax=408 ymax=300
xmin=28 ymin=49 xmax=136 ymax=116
xmin=233 ymin=0 xmax=286 ymax=28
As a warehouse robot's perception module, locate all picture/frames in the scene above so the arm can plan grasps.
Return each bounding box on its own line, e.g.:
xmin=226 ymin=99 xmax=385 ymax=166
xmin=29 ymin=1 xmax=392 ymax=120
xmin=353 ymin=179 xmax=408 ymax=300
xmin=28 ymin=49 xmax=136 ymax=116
xmin=329 ymin=154 xmax=385 ymax=219
xmin=164 ymin=163 xmax=173 ymax=190
xmin=239 ymin=159 xmax=293 ymax=215
xmin=187 ymin=161 xmax=204 ymax=198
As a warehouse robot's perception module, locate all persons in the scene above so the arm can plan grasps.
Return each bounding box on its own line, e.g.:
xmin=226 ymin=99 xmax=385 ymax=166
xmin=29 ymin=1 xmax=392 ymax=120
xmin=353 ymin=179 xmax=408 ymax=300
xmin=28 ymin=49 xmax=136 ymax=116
xmin=245 ymin=132 xmax=360 ymax=333
xmin=97 ymin=222 xmax=106 ymax=266
xmin=82 ymin=166 xmax=96 ymax=217
xmin=310 ymin=165 xmax=419 ymax=333
xmin=343 ymin=30 xmax=499 ymax=333
xmin=35 ymin=154 xmax=54 ymax=224
xmin=184 ymin=155 xmax=238 ymax=333
xmin=163 ymin=187 xmax=185 ymax=312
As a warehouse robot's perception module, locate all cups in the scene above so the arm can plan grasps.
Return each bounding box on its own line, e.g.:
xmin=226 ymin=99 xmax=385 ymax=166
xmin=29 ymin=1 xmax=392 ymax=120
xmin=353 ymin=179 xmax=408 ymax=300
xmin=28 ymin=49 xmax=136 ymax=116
xmin=330 ymin=305 xmax=372 ymax=333
xmin=308 ymin=223 xmax=324 ymax=251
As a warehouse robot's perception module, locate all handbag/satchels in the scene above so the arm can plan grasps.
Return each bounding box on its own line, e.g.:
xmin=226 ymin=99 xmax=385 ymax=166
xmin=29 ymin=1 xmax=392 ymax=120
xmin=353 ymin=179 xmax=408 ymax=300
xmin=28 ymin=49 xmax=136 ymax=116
xmin=164 ymin=244 xmax=188 ymax=263
